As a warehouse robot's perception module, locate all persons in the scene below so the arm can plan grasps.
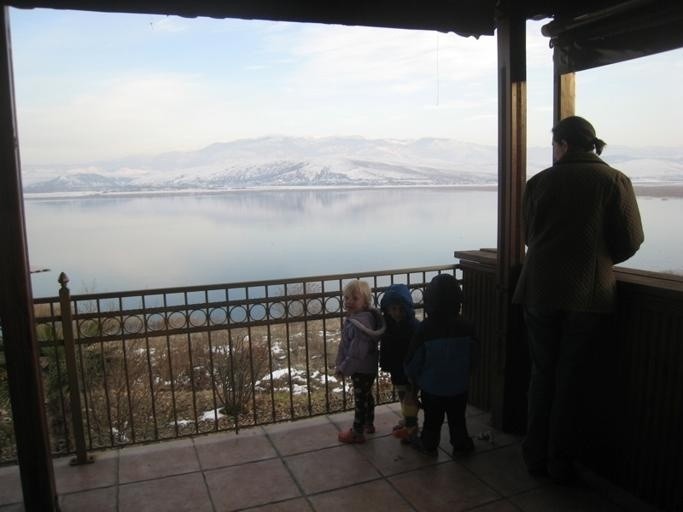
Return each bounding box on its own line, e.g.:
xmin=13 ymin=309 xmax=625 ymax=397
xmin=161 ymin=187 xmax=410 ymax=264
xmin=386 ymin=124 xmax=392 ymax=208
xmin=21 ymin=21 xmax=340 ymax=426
xmin=511 ymin=113 xmax=644 ymax=479
xmin=332 ymin=280 xmax=386 ymax=444
xmin=379 ymin=283 xmax=421 ymax=438
xmin=403 ymin=274 xmax=485 ymax=451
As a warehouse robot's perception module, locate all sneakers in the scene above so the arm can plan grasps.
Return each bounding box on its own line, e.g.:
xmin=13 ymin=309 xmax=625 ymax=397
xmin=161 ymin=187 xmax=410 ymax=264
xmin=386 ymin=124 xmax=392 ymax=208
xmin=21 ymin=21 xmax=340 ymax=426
xmin=363 ymin=423 xmax=375 ymax=433
xmin=414 ymin=435 xmax=438 ymax=457
xmin=452 ymin=442 xmax=476 ymax=455
xmin=338 ymin=428 xmax=365 ymax=443
xmin=392 ymin=419 xmax=417 ymax=441
